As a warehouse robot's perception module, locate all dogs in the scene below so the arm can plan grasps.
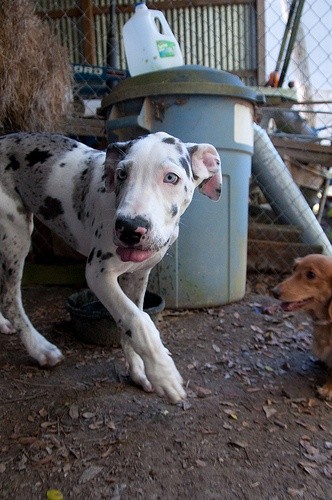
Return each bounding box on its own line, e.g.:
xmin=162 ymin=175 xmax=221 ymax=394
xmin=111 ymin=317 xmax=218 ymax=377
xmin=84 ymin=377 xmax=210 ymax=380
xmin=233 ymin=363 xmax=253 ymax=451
xmin=272 ymin=253 xmax=332 ymax=401
xmin=1 ymin=131 xmax=222 ymax=405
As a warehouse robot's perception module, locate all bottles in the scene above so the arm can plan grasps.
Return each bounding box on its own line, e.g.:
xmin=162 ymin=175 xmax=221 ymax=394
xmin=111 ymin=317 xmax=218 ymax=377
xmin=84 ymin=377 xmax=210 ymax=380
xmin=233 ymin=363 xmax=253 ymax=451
xmin=122 ymin=2 xmax=184 ymax=77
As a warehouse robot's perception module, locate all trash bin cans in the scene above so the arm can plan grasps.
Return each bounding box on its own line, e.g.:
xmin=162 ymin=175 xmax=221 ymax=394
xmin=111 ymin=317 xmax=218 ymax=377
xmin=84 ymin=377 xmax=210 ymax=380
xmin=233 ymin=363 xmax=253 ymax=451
xmin=101 ymin=65 xmax=266 ymax=308
